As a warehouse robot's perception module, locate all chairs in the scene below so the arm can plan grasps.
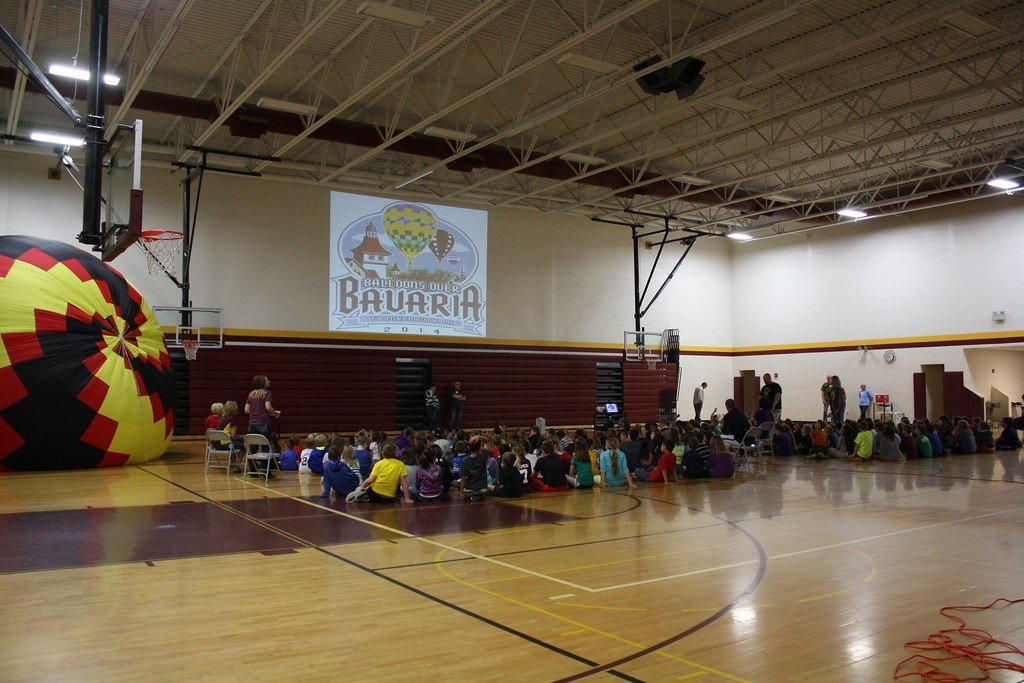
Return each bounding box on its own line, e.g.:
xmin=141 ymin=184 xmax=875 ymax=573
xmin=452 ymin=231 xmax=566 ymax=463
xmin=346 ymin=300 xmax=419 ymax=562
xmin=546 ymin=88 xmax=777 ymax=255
xmin=728 ymin=422 xmax=774 ymax=468
xmin=243 ymin=433 xmax=283 ymax=482
xmin=206 ymin=428 xmax=242 ymax=476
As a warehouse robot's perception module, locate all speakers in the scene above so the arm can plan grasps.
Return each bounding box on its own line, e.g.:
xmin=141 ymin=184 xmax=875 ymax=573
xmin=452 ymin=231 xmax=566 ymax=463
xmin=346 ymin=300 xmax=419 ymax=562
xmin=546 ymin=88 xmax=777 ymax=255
xmin=633 ymin=54 xmax=706 ymax=101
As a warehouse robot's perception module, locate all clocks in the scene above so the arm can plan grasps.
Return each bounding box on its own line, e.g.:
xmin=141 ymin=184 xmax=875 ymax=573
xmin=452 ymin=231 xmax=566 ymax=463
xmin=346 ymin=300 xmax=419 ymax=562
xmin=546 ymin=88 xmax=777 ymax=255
xmin=883 ymin=350 xmax=896 ymax=364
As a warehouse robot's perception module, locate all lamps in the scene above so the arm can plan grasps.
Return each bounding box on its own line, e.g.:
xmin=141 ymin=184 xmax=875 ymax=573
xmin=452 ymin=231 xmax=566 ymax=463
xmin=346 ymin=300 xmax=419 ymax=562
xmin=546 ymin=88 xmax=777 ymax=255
xmin=993 ymin=311 xmax=1006 ymax=321
xmin=555 ymin=51 xmax=621 ymax=76
xmin=672 ymin=173 xmax=710 ymax=188
xmin=916 ymin=157 xmax=953 ymax=173
xmin=424 ymin=127 xmax=476 ymax=143
xmin=939 ymin=7 xmax=1000 ymax=41
xmin=826 ymin=132 xmax=868 ymax=147
xmin=764 ymin=193 xmax=796 ymax=205
xmin=220 ymin=104 xmax=282 ymax=139
xmin=255 ymin=96 xmax=315 ymax=117
xmin=357 ymin=2 xmax=435 ymax=31
xmin=560 ymin=151 xmax=605 ymax=165
xmin=711 ymin=95 xmax=761 ymax=116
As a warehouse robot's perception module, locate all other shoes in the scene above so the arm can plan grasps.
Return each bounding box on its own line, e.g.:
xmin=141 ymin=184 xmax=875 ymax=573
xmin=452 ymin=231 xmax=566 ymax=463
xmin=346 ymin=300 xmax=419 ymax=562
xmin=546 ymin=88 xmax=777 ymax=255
xmin=234 ymin=467 xmax=242 ymax=474
xmin=250 ymin=472 xmax=259 ymax=477
xmin=264 ymin=473 xmax=276 ymax=479
xmin=346 ymin=486 xmax=366 ymax=503
xmin=470 ymin=495 xmax=485 ymax=501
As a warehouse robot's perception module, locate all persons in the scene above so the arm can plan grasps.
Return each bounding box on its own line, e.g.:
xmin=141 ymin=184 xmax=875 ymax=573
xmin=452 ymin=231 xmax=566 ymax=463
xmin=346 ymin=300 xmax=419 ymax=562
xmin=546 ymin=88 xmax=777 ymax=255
xmin=592 ymin=414 xmax=734 ymax=490
xmin=722 ymin=399 xmax=755 ymax=457
xmin=803 ymin=417 xmax=943 ymax=461
xmin=821 ymin=375 xmax=873 ymax=423
xmin=308 ymin=434 xmax=328 ymax=474
xmin=328 ymin=433 xmax=341 ymax=446
xmin=291 ymin=433 xmax=304 ymax=463
xmin=319 ymin=446 xmax=360 ymax=498
xmin=481 ymin=426 xmax=595 ymax=499
xmin=214 ymin=400 xmax=244 ymax=473
xmin=245 ymin=375 xmax=279 ymax=479
xmin=693 ymin=382 xmax=708 ymax=420
xmin=205 ymin=403 xmax=224 ymax=446
xmin=425 ymin=379 xmax=466 ymax=431
xmin=320 ymin=438 xmax=344 ymax=484
xmin=262 ymin=432 xmax=281 ymax=469
xmin=325 ymin=454 xmax=1024 ymax=557
xmin=308 ymin=433 xmax=316 ymax=438
xmin=750 ymin=397 xmax=804 ymax=456
xmin=299 ymin=438 xmax=314 ymax=474
xmin=349 ymin=429 xmax=415 ymax=504
xmin=1013 ymin=393 xmax=1024 ymax=431
xmin=711 ymin=408 xmax=721 ymax=435
xmin=758 ymin=373 xmax=782 ymax=420
xmin=278 ymin=439 xmax=299 ymax=470
xmin=393 ymin=426 xmax=489 ymax=502
xmin=936 ymin=416 xmax=1018 ymax=454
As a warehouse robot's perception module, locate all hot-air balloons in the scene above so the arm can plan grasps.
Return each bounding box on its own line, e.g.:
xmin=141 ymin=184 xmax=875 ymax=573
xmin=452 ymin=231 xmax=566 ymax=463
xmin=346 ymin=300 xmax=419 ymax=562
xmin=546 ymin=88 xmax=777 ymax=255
xmin=0 ymin=232 xmax=179 ymax=474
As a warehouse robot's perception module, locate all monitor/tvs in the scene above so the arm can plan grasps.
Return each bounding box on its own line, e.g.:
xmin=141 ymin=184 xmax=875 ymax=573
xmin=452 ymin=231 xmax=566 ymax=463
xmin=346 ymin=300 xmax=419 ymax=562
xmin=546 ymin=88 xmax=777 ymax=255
xmin=605 ymin=403 xmax=619 ymax=414
